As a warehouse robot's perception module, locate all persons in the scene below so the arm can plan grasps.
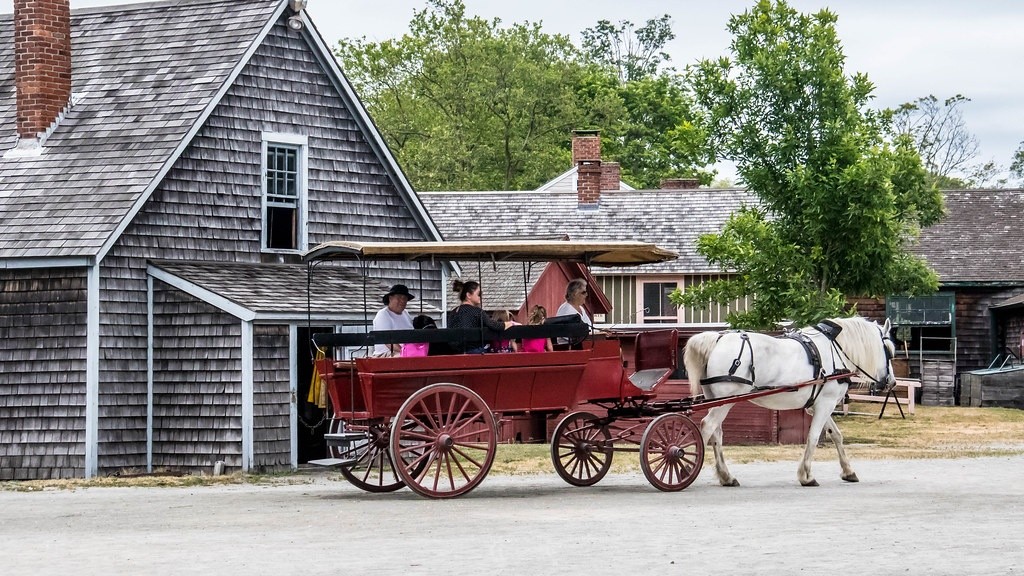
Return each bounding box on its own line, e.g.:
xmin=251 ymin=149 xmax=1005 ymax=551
xmin=372 ymin=284 xmax=555 ymax=358
xmin=890 ymin=327 xmax=904 ymax=350
xmin=555 ymin=278 xmax=624 ymax=366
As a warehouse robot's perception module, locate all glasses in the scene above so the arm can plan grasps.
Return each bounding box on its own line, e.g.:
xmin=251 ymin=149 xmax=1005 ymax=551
xmin=576 ymin=291 xmax=586 ymax=296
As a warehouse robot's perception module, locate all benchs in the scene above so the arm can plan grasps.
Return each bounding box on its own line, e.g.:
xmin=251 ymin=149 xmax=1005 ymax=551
xmin=310 ymin=315 xmax=621 ymax=412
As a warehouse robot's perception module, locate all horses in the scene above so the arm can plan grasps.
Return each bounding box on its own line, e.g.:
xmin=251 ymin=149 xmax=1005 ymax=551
xmin=682 ymin=316 xmax=896 ymax=489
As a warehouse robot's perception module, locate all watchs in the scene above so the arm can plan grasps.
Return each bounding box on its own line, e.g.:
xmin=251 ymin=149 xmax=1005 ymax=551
xmin=510 ymin=320 xmax=514 ymax=327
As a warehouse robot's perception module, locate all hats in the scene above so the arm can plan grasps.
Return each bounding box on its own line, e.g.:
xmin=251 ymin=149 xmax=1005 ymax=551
xmin=383 ymin=285 xmax=415 ymax=305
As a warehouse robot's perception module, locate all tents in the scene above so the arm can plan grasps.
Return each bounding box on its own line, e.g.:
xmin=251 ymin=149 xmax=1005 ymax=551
xmin=291 ymin=236 xmax=678 ymax=266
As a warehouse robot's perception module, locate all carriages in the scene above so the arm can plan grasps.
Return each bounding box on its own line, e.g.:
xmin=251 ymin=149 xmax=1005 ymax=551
xmin=304 ymin=240 xmax=900 ymax=500
